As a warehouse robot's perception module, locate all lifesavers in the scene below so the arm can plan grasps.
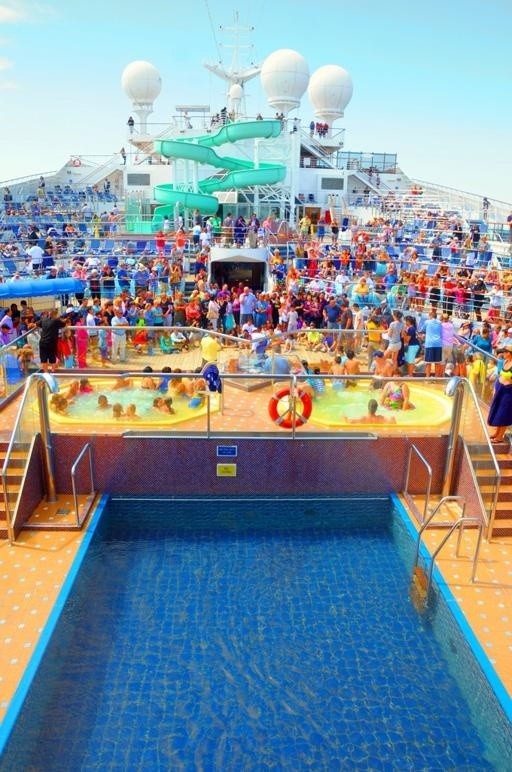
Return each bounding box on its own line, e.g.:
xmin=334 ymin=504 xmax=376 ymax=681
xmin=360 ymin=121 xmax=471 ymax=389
xmin=269 ymin=388 xmax=311 ymax=428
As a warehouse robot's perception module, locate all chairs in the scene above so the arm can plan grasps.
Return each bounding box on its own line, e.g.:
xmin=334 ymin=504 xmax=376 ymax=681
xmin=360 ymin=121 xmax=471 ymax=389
xmin=0 ymin=183 xmax=511 ymax=299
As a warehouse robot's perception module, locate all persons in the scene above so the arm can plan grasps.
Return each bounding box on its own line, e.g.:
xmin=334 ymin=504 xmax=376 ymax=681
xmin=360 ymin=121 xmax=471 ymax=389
xmin=120 ymin=146 xmax=127 ymax=165
xmin=182 ymin=107 xmax=331 ymax=139
xmin=128 ymin=116 xmax=134 ymax=136
xmin=1 ymin=165 xmax=511 ymax=444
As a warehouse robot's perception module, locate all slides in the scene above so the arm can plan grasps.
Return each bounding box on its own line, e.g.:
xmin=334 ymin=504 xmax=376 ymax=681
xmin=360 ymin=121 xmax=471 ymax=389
xmin=151 ymin=120 xmax=283 ymax=232
